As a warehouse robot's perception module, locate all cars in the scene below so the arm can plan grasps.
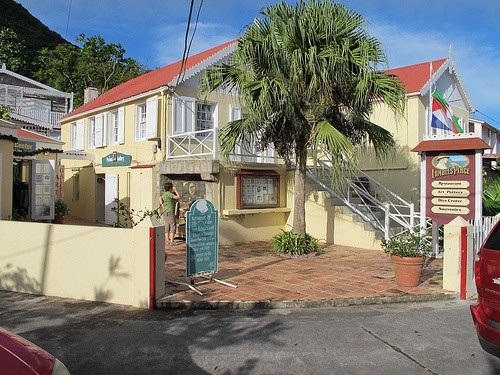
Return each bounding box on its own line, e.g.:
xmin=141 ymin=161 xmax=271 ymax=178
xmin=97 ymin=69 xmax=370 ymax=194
xmin=0 ymin=327 xmax=72 ymax=375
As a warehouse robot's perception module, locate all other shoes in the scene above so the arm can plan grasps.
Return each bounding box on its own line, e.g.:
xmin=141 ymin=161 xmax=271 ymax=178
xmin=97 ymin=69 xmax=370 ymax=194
xmin=170 ymin=242 xmax=179 ymax=245
xmin=166 ymin=242 xmax=170 ymax=245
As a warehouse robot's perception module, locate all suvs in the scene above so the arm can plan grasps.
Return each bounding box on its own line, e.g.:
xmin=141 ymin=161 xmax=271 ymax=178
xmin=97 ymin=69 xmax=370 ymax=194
xmin=469 ymin=217 xmax=500 ymax=359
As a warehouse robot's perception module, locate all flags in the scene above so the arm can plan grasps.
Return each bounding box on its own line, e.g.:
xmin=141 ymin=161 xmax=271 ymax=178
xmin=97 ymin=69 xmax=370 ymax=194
xmin=431 ymin=85 xmax=464 ymax=134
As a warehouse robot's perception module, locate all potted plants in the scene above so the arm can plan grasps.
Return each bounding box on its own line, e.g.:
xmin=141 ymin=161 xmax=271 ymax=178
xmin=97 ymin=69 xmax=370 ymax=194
xmin=41 ymin=198 xmax=72 ymax=224
xmin=379 ymin=224 xmax=434 ymax=287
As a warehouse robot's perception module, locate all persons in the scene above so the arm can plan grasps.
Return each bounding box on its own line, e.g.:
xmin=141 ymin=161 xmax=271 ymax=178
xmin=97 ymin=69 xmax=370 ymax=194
xmin=159 ymin=182 xmax=180 ymax=246
